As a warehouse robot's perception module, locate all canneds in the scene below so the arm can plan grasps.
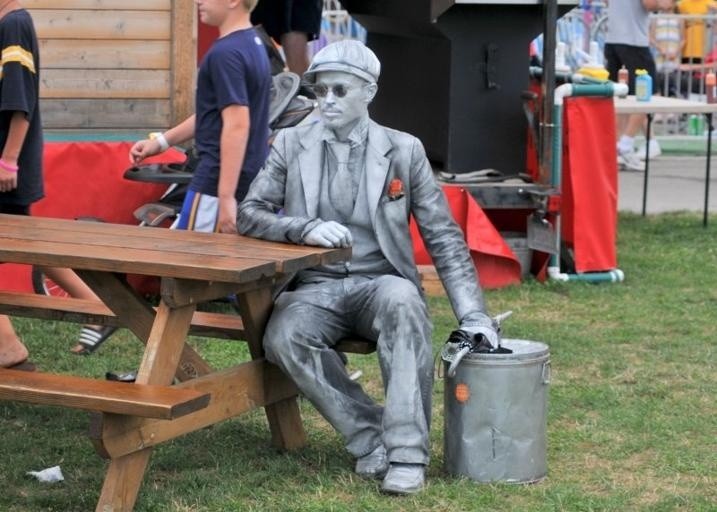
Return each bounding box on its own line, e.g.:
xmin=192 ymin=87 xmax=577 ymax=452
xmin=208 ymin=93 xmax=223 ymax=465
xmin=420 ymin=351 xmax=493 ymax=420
xmin=689 ymin=115 xmax=704 ymax=136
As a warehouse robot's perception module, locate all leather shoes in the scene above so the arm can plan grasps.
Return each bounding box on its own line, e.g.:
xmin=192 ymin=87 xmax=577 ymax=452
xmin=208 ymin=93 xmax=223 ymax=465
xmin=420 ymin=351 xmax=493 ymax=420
xmin=353 ymin=439 xmax=389 ymax=476
xmin=380 ymin=458 xmax=428 ymax=496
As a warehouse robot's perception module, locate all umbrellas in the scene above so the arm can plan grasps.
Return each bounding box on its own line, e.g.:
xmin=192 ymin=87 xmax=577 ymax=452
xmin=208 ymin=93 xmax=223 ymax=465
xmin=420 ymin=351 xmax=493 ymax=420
xmin=437 ymin=302 xmax=512 ymax=382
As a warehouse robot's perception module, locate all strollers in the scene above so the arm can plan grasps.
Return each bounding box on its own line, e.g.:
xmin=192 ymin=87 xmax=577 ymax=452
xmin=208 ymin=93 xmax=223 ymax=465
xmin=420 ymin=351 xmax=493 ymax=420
xmin=33 ymin=72 xmax=319 ymax=318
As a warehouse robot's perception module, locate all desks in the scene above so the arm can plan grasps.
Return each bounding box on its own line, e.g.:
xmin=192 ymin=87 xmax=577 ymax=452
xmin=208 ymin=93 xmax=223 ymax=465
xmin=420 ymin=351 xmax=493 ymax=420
xmin=0 ymin=212 xmax=353 ymax=512
xmin=612 ymin=94 xmax=717 ymax=228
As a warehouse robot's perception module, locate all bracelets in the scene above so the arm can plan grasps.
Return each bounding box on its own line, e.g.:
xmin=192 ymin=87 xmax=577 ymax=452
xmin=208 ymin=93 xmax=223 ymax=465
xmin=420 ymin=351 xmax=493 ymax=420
xmin=0 ymin=161 xmax=22 ymax=175
xmin=147 ymin=128 xmax=171 ymax=154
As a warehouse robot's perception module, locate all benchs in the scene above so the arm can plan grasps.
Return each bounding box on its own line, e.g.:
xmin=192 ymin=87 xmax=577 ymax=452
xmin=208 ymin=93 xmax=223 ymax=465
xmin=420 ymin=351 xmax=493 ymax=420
xmin=0 ymin=366 xmax=210 ymax=512
xmin=0 ymin=290 xmax=377 ymax=451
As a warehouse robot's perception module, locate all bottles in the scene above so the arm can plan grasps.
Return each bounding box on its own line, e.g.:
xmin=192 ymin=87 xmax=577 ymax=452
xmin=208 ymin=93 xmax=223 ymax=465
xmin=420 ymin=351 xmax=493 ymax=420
xmin=704 ymin=69 xmax=717 ymax=106
xmin=618 ymin=64 xmax=630 ymax=97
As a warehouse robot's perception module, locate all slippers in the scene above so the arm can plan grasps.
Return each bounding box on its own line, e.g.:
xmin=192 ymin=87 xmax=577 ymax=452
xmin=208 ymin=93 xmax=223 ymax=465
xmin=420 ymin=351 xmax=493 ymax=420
xmin=71 ymin=311 xmax=121 ymax=355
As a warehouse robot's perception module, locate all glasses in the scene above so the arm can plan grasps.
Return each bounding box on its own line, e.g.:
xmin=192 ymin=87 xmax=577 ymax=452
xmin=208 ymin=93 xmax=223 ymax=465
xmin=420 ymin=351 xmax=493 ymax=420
xmin=313 ymin=82 xmax=366 ymax=97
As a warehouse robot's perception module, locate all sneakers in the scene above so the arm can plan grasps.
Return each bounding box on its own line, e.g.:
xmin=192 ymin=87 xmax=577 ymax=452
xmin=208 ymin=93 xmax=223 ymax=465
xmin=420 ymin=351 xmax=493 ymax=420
xmin=637 ymin=137 xmax=663 ymax=160
xmin=616 ymin=140 xmax=647 ymax=172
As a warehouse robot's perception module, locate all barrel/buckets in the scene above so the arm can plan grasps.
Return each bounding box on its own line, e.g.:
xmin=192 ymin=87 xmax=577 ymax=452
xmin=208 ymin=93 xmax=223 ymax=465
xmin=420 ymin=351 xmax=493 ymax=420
xmin=497 ymin=230 xmax=535 ymax=275
xmin=438 ymin=337 xmax=552 ymax=485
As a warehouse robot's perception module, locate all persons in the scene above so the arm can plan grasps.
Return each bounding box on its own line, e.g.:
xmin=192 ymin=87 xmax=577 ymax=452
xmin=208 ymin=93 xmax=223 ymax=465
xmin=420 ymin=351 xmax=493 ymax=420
xmin=121 ymin=2 xmax=276 ymax=241
xmin=231 ymin=39 xmax=500 ymax=496
xmin=0 ymin=1 xmax=122 ymax=375
xmin=554 ymin=0 xmax=716 ymax=176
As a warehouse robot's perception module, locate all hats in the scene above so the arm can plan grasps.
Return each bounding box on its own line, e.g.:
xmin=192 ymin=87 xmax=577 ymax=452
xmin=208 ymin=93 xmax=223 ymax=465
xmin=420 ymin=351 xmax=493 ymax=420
xmin=302 ymin=39 xmax=382 ymax=85
